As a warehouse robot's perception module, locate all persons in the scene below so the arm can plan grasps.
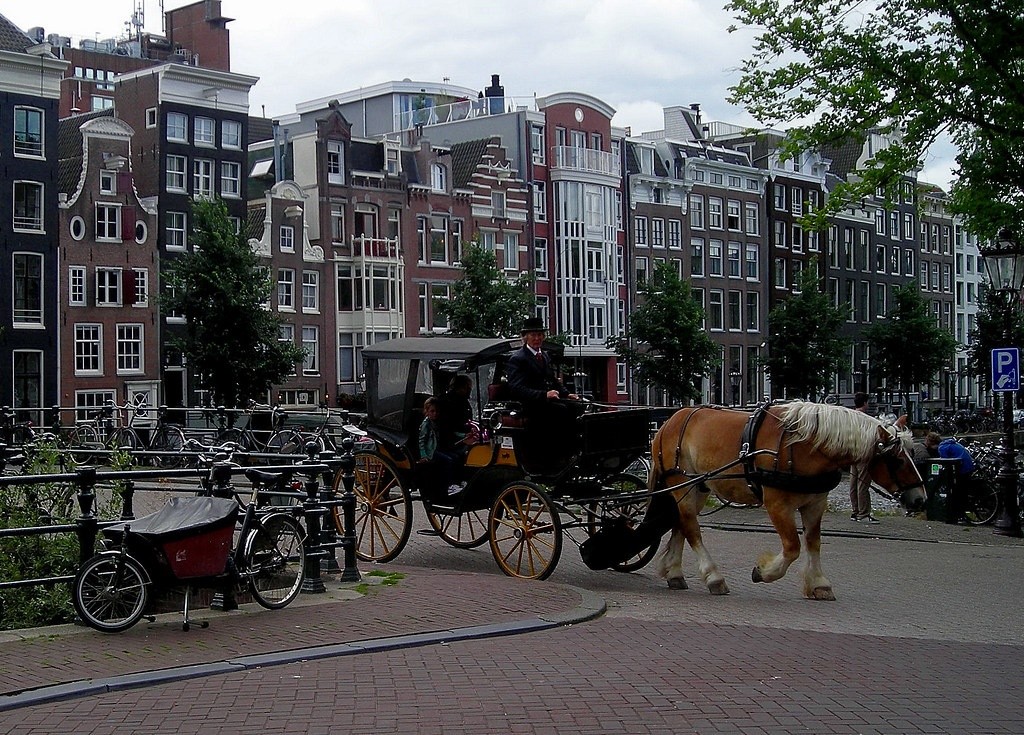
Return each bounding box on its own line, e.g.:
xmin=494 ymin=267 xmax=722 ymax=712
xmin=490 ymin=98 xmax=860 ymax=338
xmin=505 ymin=318 xmax=580 ymax=465
xmin=851 ymin=392 xmax=881 ymax=523
xmin=419 ymin=396 xmax=467 ymax=497
xmin=440 ymin=374 xmax=472 ymax=455
xmin=925 ymin=432 xmax=976 ymax=523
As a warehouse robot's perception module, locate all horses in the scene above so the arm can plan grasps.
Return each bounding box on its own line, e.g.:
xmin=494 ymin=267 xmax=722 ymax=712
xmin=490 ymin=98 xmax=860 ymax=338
xmin=649 ymin=399 xmax=929 ymax=601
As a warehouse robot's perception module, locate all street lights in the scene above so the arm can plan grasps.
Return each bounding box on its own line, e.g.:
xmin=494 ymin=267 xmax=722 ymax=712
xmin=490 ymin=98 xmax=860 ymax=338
xmin=984 ymin=232 xmax=1024 ymax=541
xmin=728 ymin=371 xmax=743 ymax=406
xmin=948 ymin=370 xmax=959 ymax=411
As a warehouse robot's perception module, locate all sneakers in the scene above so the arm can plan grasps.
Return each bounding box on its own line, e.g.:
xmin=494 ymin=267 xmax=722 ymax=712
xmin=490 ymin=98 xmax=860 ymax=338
xmin=850 ymin=513 xmax=857 ymax=521
xmin=857 ymin=515 xmax=880 ymax=523
xmin=448 ymin=484 xmax=464 ymax=496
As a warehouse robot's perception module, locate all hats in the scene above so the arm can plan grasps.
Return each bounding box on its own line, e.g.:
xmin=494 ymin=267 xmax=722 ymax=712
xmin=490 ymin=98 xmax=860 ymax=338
xmin=519 ymin=318 xmax=548 ymax=332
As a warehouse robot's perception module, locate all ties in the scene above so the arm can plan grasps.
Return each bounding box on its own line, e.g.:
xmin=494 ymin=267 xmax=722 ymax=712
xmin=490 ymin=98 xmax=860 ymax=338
xmin=536 ymin=354 xmax=543 ymax=363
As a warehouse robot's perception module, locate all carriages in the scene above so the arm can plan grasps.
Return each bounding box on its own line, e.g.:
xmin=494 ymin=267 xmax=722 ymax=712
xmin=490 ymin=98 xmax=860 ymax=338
xmin=327 ymin=334 xmax=932 ymax=603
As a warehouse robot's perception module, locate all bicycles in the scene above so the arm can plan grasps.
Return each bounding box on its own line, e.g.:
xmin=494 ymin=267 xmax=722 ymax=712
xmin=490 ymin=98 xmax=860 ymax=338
xmin=0 ymin=390 xmax=378 ymax=635
xmin=901 ymin=397 xmax=1024 ymax=526
